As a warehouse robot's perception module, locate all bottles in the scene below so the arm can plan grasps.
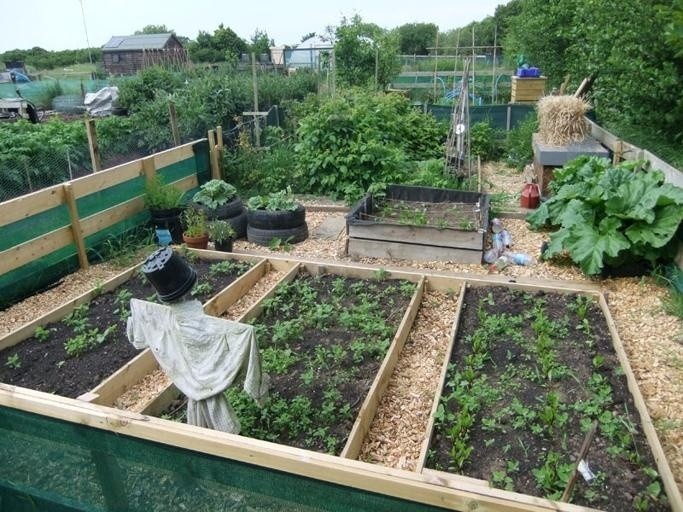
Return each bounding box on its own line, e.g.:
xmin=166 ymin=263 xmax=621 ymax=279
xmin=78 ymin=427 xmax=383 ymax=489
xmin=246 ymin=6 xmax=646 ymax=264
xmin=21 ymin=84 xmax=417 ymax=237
xmin=483 ymin=217 xmax=533 ymax=274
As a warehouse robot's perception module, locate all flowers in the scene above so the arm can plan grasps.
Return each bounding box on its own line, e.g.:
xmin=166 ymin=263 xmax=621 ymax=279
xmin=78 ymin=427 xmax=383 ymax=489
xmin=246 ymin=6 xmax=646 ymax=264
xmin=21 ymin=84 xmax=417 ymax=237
xmin=180 ymin=202 xmax=209 ymax=238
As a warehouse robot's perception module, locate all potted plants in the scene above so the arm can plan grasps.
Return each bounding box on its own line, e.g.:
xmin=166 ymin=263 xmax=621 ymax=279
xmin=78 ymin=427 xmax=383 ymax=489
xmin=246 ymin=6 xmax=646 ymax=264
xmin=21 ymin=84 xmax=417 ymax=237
xmin=141 ymin=168 xmax=183 ymax=229
xmin=206 ymin=215 xmax=236 ymax=252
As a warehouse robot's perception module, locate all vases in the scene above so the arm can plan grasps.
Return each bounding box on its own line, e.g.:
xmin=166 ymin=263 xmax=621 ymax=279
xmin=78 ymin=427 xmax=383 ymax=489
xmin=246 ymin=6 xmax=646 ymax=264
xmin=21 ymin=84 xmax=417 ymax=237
xmin=180 ymin=231 xmax=209 ymax=252
